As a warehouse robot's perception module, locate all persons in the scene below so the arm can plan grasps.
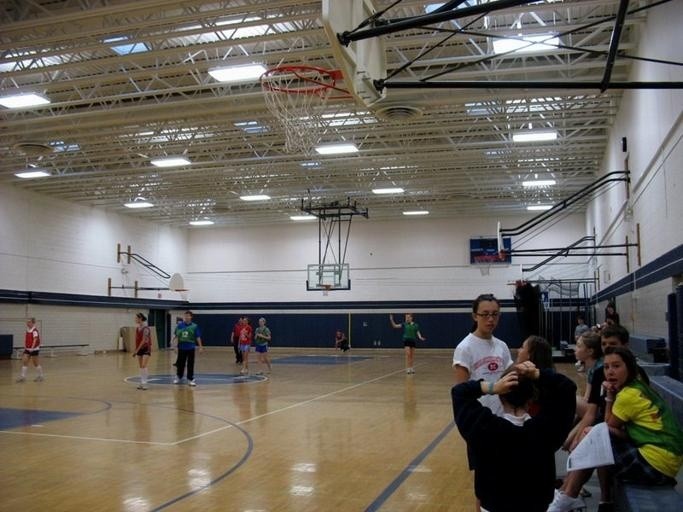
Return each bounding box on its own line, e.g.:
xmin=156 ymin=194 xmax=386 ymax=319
xmin=237 ymin=317 xmax=252 ymax=374
xmin=231 ymin=316 xmax=244 ymax=364
xmin=390 ymin=313 xmax=426 ymax=374
xmin=254 ymin=317 xmax=272 ymax=375
xmin=16 ymin=318 xmax=44 ymax=382
xmin=335 ymin=330 xmax=349 ymax=352
xmin=173 ymin=318 xmax=183 ymax=365
xmin=132 ymin=313 xmax=152 ymax=389
xmin=170 ymin=311 xmax=203 ymax=386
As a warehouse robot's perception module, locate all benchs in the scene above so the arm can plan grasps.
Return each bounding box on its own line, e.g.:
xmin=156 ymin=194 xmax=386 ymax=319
xmin=12 ymin=344 xmax=88 ymax=359
xmin=598 ymin=375 xmax=683 ymax=512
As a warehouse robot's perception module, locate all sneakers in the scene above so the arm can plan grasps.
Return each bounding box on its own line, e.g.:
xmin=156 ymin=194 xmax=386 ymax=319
xmin=188 ymin=379 xmax=197 ymax=387
xmin=547 ymin=483 xmax=593 ymax=511
xmin=33 ymin=377 xmax=44 ymax=383
xmin=240 ymin=370 xmax=272 ymax=376
xmin=136 ymin=383 xmax=149 ymax=390
xmin=15 ymin=376 xmax=25 ymax=382
xmin=406 ymin=368 xmax=415 ymax=374
xmin=575 ymin=360 xmax=584 ymax=372
xmin=173 ymin=376 xmax=182 ymax=385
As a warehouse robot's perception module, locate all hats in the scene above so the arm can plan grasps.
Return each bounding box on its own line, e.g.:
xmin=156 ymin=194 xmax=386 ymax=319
xmin=136 ymin=312 xmax=148 ymax=321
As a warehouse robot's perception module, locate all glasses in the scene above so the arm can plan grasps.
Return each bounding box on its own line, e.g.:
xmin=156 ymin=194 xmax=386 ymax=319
xmin=476 ymin=312 xmax=500 ymax=317
